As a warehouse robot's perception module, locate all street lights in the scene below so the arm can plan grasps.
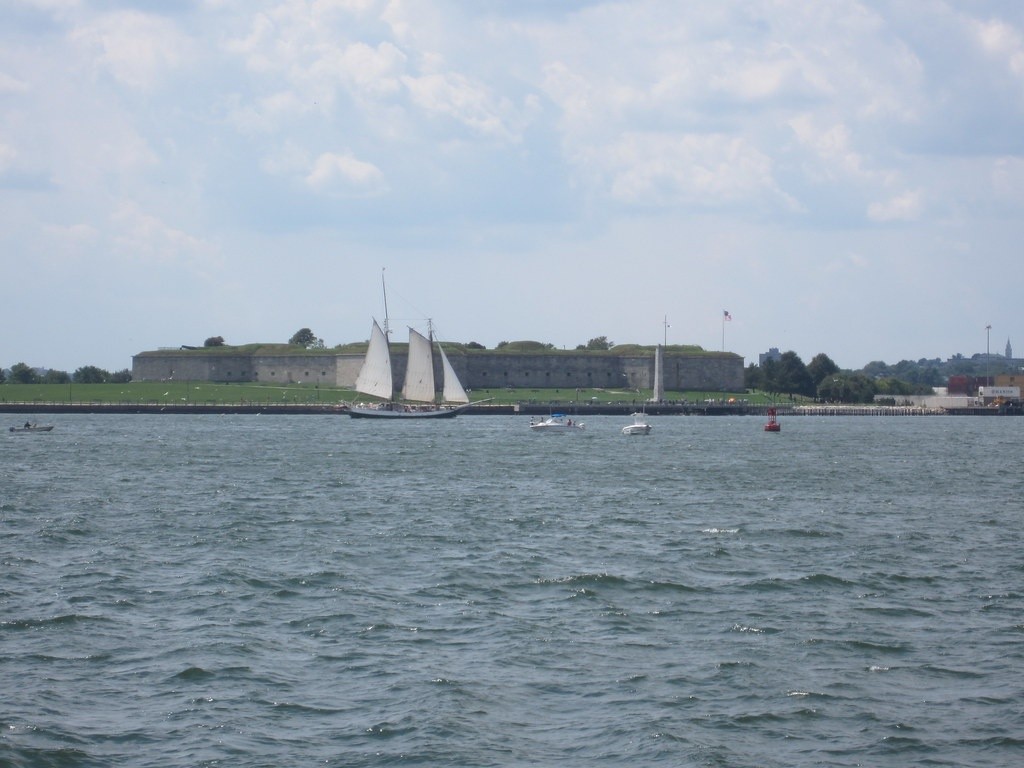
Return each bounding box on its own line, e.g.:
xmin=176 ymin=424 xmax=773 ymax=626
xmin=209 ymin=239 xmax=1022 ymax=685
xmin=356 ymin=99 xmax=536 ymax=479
xmin=985 ymin=325 xmax=992 ymax=388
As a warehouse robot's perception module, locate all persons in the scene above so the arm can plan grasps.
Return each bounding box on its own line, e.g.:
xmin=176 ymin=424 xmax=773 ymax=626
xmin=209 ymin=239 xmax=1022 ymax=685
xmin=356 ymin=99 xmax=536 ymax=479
xmin=24 ymin=421 xmax=31 ymax=429
xmin=531 ymin=416 xmax=534 ymax=425
xmin=567 ymin=419 xmax=571 ymax=426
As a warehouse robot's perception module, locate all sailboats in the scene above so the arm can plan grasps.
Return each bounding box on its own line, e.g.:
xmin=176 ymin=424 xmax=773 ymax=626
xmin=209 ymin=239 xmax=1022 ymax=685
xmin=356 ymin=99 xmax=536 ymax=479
xmin=343 ymin=266 xmax=497 ymax=419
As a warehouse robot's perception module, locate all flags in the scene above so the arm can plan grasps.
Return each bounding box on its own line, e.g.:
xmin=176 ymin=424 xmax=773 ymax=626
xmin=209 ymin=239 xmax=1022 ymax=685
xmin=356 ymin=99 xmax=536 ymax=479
xmin=725 ymin=310 xmax=731 ymax=321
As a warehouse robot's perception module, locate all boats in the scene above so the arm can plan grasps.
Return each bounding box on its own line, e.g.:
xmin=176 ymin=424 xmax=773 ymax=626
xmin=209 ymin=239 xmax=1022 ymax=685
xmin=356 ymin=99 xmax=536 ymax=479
xmin=621 ymin=412 xmax=652 ymax=435
xmin=764 ymin=407 xmax=780 ymax=432
xmin=8 ymin=422 xmax=54 ymax=432
xmin=529 ymin=414 xmax=586 ymax=433
